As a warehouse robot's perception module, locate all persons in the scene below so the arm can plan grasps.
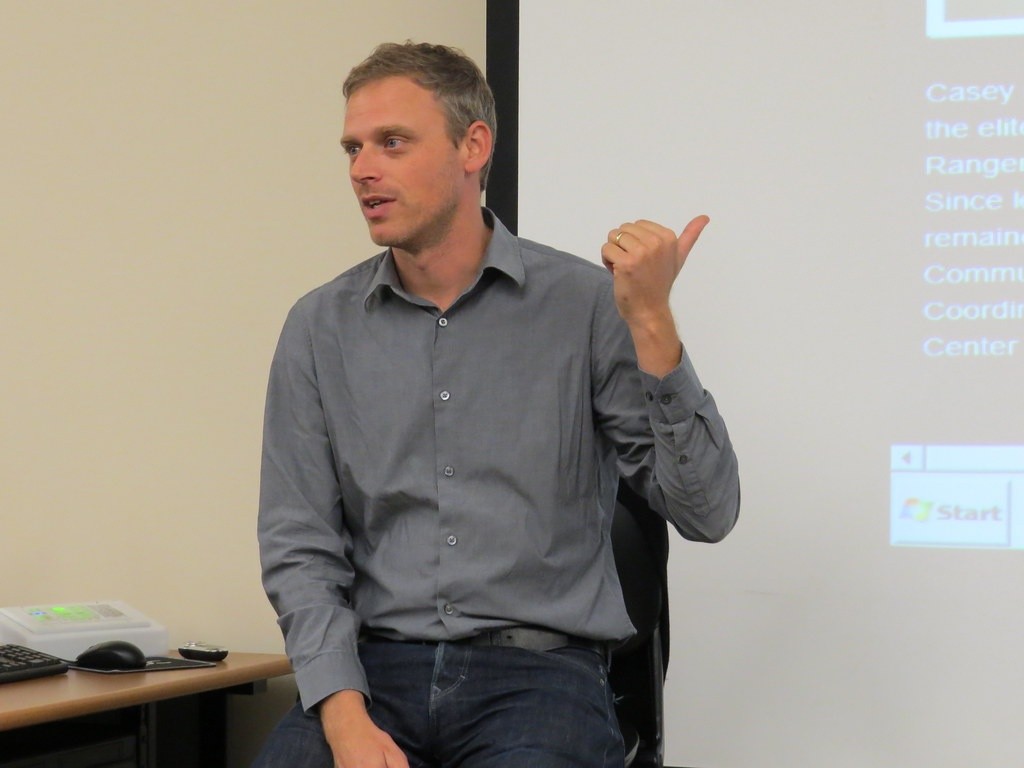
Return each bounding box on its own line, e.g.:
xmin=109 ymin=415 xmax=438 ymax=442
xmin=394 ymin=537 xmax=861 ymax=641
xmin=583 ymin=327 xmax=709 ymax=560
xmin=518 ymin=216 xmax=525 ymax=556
xmin=254 ymin=43 xmax=744 ymax=768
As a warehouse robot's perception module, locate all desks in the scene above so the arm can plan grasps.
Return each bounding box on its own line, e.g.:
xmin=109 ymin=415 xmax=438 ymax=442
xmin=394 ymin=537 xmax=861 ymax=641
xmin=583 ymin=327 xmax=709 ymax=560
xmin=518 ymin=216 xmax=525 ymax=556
xmin=0 ymin=651 xmax=293 ymax=768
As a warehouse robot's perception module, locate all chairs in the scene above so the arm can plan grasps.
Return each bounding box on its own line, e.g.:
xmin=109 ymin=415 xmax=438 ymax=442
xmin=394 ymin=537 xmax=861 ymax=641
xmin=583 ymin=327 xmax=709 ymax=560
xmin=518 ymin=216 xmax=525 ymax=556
xmin=612 ymin=477 xmax=673 ymax=768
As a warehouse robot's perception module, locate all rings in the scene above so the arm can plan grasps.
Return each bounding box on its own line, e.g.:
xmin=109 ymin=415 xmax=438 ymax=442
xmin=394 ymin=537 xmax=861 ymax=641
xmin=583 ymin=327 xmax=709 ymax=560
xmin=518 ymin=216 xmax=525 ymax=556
xmin=615 ymin=232 xmax=625 ymax=246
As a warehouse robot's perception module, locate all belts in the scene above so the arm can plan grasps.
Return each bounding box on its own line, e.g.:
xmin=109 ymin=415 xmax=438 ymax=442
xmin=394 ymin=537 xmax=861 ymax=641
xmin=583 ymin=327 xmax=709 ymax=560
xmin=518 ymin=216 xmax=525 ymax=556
xmin=468 ymin=626 xmax=606 ymax=662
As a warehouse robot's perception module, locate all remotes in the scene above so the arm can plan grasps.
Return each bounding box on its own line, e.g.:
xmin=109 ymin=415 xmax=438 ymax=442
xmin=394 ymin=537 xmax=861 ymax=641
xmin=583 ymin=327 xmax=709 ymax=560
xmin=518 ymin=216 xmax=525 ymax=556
xmin=178 ymin=646 xmax=229 ymax=663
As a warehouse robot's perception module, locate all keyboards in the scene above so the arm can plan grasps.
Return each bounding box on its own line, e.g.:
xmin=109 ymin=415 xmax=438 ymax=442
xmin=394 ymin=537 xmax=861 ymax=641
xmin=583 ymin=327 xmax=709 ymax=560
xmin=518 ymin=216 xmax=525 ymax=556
xmin=0 ymin=643 xmax=69 ymax=684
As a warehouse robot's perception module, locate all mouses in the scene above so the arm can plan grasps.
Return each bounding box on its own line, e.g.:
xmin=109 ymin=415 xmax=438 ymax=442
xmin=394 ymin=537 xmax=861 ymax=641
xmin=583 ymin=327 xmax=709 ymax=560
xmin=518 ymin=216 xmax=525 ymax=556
xmin=76 ymin=641 xmax=146 ymax=671
